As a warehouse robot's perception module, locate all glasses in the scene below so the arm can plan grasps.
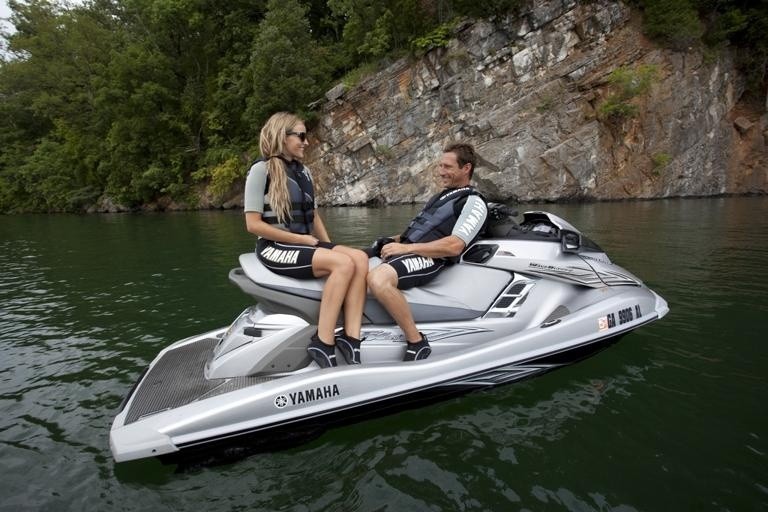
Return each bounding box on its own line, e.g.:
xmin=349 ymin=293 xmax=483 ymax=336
xmin=287 ymin=132 xmax=306 ymax=142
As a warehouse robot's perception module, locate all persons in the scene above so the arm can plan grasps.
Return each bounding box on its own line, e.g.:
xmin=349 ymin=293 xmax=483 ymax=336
xmin=244 ymin=111 xmax=369 ymax=369
xmin=367 ymin=143 xmax=490 ymax=361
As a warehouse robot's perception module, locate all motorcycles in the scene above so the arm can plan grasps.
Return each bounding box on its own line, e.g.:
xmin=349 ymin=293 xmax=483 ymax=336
xmin=107 ymin=201 xmax=669 ymax=463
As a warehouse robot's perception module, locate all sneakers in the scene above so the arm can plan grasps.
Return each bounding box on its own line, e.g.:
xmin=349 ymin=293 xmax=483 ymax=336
xmin=307 ymin=329 xmax=366 ymax=368
xmin=404 ymin=332 xmax=432 ymax=360
